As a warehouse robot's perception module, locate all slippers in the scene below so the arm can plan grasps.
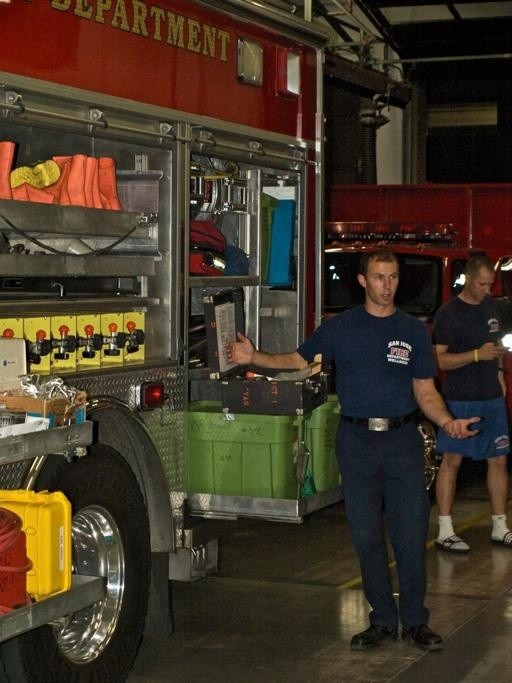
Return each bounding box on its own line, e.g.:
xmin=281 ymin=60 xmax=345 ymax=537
xmin=435 ymin=534 xmax=471 ymax=552
xmin=492 ymin=530 xmax=512 ymax=546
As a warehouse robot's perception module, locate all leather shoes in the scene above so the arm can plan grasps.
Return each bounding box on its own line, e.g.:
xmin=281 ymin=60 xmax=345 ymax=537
xmin=350 ymin=624 xmax=398 ymax=651
xmin=401 ymin=623 xmax=444 ymax=651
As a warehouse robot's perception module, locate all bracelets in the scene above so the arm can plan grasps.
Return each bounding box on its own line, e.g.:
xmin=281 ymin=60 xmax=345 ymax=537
xmin=473 ymin=348 xmax=479 ymax=362
xmin=497 ymin=366 xmax=507 ymax=374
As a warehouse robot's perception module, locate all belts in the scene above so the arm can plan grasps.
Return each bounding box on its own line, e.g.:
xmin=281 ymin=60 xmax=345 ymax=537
xmin=341 ymin=413 xmax=411 ymax=432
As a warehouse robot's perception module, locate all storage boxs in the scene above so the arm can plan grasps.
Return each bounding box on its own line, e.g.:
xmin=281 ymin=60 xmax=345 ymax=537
xmin=1 ymin=390 xmax=86 ymax=427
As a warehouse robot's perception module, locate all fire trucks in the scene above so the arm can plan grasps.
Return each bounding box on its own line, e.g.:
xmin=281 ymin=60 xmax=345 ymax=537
xmin=1 ymin=0 xmax=347 ymax=683
xmin=325 ymin=178 xmax=512 ymax=495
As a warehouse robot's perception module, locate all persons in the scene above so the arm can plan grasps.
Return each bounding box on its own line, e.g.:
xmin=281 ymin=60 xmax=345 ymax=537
xmin=430 ymin=250 xmax=511 ymax=553
xmin=222 ymin=243 xmax=481 ymax=652
